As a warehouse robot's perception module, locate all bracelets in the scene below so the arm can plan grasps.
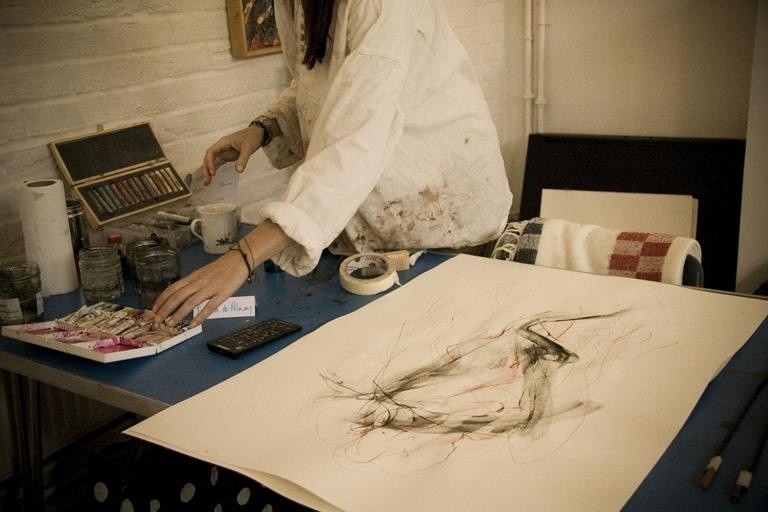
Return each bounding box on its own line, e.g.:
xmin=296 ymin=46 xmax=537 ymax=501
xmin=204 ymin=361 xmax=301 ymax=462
xmin=229 ymin=231 xmax=255 ymax=284
xmin=242 ymin=121 xmax=271 ymax=147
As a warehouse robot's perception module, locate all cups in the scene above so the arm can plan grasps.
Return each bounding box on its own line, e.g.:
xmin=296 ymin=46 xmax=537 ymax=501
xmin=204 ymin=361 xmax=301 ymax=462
xmin=78 ymin=245 xmax=125 ymax=301
xmin=191 ymin=202 xmax=239 ymax=254
xmin=0 ymin=261 xmax=45 ymax=325
xmin=127 ymin=237 xmax=181 ymax=310
xmin=66 ymin=198 xmax=91 ymax=273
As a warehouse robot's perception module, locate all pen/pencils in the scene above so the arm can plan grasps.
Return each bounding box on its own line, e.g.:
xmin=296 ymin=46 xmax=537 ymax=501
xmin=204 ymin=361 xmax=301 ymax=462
xmin=86 ymin=167 xmax=183 ymax=214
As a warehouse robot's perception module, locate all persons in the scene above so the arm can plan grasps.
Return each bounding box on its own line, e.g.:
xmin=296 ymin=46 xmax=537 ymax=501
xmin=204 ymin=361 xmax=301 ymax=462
xmin=145 ymin=1 xmax=517 ymax=335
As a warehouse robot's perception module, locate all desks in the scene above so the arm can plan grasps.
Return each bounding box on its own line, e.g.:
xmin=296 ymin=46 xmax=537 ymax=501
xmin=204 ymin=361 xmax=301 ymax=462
xmin=1 ymin=212 xmax=768 ymax=512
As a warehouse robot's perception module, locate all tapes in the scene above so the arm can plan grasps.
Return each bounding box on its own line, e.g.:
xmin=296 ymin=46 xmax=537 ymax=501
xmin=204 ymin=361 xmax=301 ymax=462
xmin=338 ymin=252 xmax=402 ymax=296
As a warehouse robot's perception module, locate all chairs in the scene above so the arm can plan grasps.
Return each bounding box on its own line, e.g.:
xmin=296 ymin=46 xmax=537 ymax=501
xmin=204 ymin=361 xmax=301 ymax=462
xmin=492 ymin=217 xmax=704 ymax=290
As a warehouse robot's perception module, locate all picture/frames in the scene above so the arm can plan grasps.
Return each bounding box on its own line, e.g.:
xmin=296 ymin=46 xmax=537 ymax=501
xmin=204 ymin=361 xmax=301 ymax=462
xmin=225 ymin=0 xmax=283 ymax=60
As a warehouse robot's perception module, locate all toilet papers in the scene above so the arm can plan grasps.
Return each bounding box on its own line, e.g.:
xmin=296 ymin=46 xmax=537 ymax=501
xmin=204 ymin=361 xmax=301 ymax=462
xmin=18 ymin=178 xmax=80 ymax=297
